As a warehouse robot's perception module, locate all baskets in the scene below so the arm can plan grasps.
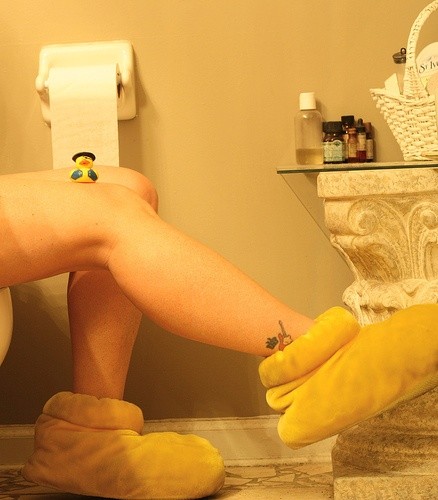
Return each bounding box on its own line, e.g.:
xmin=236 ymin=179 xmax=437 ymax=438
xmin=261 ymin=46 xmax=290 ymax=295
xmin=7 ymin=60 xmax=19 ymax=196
xmin=369 ymin=1 xmax=438 ymax=161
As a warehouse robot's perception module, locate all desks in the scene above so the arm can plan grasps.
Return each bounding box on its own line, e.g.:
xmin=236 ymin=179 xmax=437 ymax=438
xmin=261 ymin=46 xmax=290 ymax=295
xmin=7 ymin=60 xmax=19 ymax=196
xmin=278 ymin=161 xmax=438 ymax=500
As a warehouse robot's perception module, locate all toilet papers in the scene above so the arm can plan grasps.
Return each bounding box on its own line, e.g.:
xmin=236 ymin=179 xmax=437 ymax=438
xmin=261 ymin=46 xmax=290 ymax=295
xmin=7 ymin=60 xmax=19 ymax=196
xmin=48 ymin=61 xmax=121 ymax=171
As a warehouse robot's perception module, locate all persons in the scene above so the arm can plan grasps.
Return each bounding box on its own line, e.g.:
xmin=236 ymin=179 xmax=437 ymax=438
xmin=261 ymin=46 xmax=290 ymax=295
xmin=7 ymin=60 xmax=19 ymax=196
xmin=0 ymin=166 xmax=438 ymax=500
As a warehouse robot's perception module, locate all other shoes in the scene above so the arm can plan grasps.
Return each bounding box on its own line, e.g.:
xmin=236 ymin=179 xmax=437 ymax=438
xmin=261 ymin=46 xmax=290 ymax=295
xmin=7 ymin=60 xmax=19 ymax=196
xmin=20 ymin=392 xmax=225 ymax=499
xmin=258 ymin=306 xmax=438 ymax=450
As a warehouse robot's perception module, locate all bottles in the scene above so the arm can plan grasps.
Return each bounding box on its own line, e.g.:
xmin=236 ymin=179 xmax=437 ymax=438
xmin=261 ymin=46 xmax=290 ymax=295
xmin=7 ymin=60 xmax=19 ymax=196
xmin=346 ymin=128 xmax=357 ymax=162
xmin=356 ymin=118 xmax=365 ymax=163
xmin=365 ymin=132 xmax=372 ymax=162
xmin=294 ymin=93 xmax=323 ymax=166
xmin=322 ymin=120 xmax=346 ymax=162
